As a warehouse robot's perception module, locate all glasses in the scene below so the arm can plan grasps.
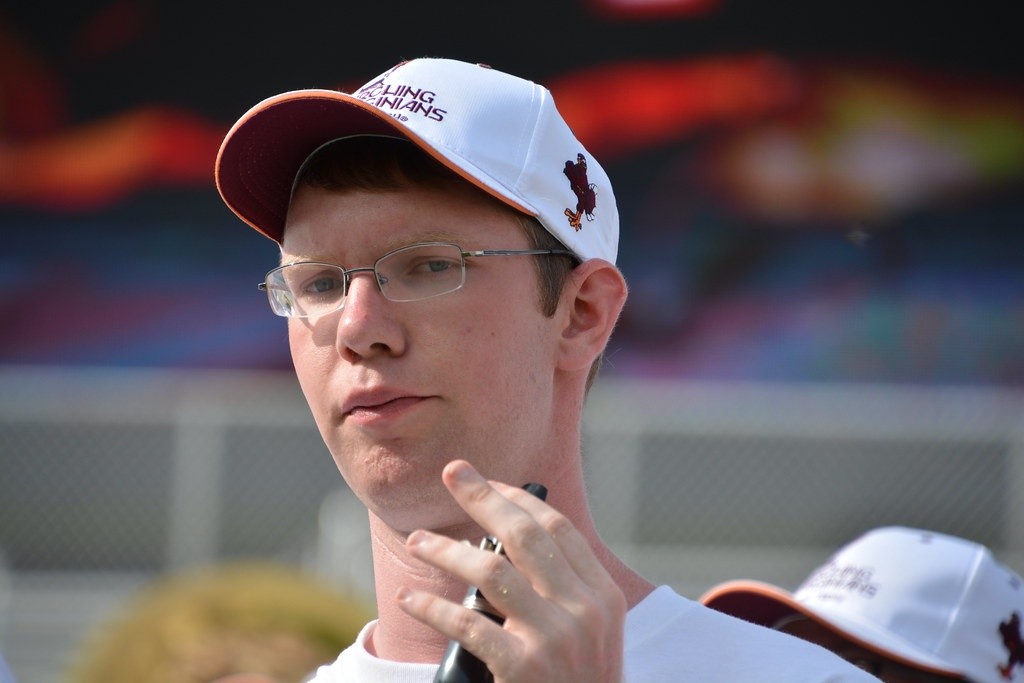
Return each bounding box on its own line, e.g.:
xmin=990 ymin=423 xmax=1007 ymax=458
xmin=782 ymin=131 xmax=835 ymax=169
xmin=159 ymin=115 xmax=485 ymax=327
xmin=257 ymin=242 xmax=582 ymax=317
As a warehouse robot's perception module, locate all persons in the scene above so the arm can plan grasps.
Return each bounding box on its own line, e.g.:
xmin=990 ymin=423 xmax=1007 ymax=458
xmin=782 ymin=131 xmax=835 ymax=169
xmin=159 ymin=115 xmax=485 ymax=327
xmin=694 ymin=525 xmax=1023 ymax=683
xmin=215 ymin=59 xmax=880 ymax=683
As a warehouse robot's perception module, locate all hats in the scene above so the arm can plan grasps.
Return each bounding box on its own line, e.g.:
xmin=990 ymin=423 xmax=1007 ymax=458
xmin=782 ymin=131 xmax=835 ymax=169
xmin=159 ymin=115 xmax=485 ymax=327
xmin=703 ymin=527 xmax=1024 ymax=683
xmin=214 ymin=56 xmax=620 ymax=273
